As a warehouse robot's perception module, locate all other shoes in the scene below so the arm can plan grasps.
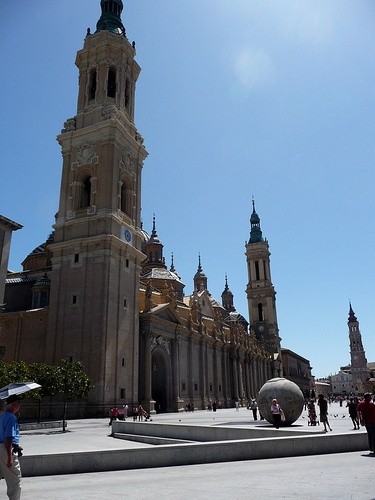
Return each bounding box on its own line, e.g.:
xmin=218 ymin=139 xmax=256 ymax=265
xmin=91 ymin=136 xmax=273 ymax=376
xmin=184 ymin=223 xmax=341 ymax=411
xmin=323 ymin=429 xmax=328 ymax=432
xmin=330 ymin=428 xmax=332 ymax=431
xmin=369 ymin=452 xmax=375 ymax=457
xmin=263 ymin=419 xmax=264 ymax=420
xmin=358 ymin=427 xmax=360 ymax=430
xmin=260 ymin=419 xmax=262 ymax=420
xmin=276 ymin=426 xmax=279 ymax=429
xmin=254 ymin=419 xmax=257 ymax=421
xmin=354 ymin=427 xmax=356 ymax=430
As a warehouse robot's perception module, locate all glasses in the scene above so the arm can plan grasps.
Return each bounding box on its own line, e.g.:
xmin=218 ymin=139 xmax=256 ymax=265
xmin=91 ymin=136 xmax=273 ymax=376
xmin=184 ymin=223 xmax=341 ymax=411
xmin=252 ymin=399 xmax=255 ymax=402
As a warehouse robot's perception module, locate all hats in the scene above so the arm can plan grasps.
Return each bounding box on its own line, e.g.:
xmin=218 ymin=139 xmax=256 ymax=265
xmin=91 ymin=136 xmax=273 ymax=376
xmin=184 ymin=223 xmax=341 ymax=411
xmin=6 ymin=395 xmax=24 ymax=405
xmin=272 ymin=399 xmax=278 ymax=402
xmin=318 ymin=393 xmax=324 ymax=398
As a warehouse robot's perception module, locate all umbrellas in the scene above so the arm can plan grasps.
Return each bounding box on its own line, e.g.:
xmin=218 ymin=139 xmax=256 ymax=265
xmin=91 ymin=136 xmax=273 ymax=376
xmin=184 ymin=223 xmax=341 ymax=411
xmin=0 ymin=380 xmax=42 ymax=400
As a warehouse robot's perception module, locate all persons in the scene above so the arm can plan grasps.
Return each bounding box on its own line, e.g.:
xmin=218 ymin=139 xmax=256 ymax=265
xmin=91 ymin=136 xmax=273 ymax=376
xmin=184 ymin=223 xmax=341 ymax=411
xmin=109 ymin=391 xmax=375 ymax=430
xmin=271 ymin=399 xmax=283 ymax=429
xmin=317 ymin=394 xmax=332 ymax=431
xmin=358 ymin=393 xmax=375 ymax=456
xmin=0 ymin=394 xmax=23 ymax=500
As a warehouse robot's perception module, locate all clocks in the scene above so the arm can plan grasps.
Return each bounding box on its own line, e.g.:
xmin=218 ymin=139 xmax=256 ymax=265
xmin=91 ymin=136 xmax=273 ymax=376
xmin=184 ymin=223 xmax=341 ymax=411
xmin=124 ymin=230 xmax=132 ymax=243
xmin=259 ymin=326 xmax=264 ymax=333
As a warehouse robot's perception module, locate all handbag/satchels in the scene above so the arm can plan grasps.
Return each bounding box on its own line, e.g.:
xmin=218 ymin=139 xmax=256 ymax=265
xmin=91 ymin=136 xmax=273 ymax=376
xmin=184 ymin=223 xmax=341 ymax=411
xmin=280 ymin=409 xmax=286 ymax=421
